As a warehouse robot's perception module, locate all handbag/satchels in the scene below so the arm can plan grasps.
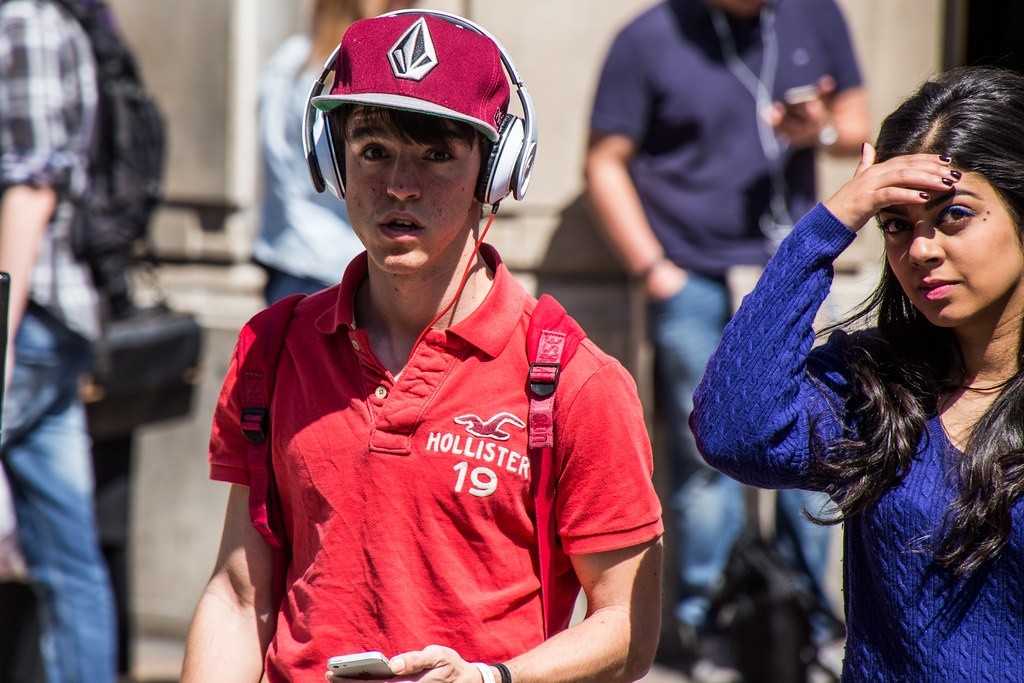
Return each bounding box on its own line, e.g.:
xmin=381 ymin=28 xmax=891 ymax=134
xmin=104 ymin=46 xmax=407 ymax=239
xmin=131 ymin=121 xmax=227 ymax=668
xmin=90 ymin=302 xmax=203 ymax=423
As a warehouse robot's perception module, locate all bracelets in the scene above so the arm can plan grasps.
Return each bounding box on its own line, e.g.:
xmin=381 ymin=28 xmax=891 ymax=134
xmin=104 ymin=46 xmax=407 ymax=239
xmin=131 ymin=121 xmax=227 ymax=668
xmin=817 ymin=116 xmax=838 ymax=152
xmin=473 ymin=660 xmax=496 ymax=683
xmin=489 ymin=663 xmax=512 ymax=683
xmin=638 ymin=256 xmax=666 ymax=284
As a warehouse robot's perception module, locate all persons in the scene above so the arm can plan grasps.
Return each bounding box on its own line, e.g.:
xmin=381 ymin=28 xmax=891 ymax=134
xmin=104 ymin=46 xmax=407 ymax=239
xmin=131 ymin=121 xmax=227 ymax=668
xmin=688 ymin=68 xmax=1022 ymax=683
xmin=1 ymin=1 xmax=163 ymax=682
xmin=188 ymin=13 xmax=664 ymax=683
xmin=245 ymin=0 xmax=426 ymax=309
xmin=589 ymin=0 xmax=874 ymax=682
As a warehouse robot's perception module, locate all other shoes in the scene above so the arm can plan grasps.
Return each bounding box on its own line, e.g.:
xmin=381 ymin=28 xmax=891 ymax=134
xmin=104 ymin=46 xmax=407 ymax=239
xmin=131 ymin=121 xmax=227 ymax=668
xmin=807 ymin=636 xmax=844 ymax=683
xmin=681 ymin=623 xmax=745 ymax=683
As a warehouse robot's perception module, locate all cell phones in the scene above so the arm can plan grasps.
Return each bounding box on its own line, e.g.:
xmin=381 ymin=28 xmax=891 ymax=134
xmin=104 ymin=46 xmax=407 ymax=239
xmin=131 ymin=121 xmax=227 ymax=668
xmin=328 ymin=652 xmax=396 ymax=680
xmin=785 ymin=86 xmax=820 ymax=112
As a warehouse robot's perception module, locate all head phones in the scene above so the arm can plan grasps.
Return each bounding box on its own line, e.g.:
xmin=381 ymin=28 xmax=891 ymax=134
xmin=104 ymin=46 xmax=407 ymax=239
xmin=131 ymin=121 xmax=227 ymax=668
xmin=302 ymin=8 xmax=538 ymax=204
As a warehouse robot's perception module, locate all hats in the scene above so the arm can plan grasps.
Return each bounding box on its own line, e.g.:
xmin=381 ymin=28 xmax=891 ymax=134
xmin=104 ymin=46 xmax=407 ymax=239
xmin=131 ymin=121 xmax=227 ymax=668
xmin=310 ymin=14 xmax=511 ymax=146
